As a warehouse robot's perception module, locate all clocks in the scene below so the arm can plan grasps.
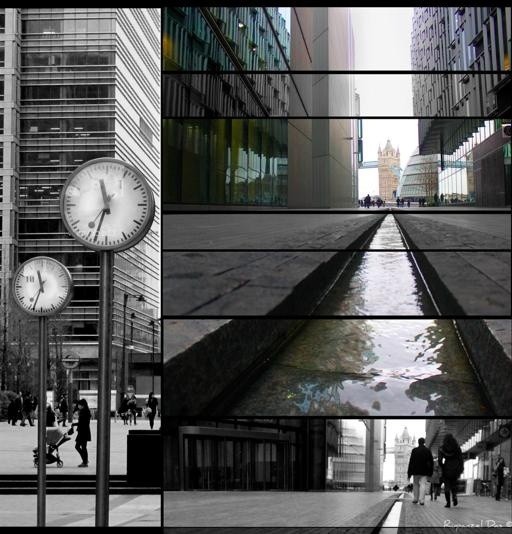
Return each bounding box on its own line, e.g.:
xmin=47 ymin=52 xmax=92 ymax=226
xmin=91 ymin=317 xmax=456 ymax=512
xmin=11 ymin=255 xmax=74 ymax=319
xmin=59 ymin=156 xmax=156 ymax=254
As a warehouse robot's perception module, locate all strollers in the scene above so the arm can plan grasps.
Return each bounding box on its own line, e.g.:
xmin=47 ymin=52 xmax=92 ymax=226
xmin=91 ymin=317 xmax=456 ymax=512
xmin=32 ymin=427 xmax=74 ymax=469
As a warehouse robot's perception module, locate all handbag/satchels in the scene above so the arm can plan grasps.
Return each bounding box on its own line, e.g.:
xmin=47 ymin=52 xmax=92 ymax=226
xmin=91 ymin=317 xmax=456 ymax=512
xmin=142 ymin=407 xmax=152 ymax=417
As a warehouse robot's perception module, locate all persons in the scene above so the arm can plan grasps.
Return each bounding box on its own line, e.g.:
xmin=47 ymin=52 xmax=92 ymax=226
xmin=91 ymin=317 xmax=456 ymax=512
xmin=118 ymin=391 xmax=161 ymax=430
xmin=424 ymin=454 xmax=444 ymax=502
xmin=406 ymin=438 xmax=433 ymax=506
xmin=357 ymin=194 xmax=386 ymax=207
xmin=494 ymin=453 xmax=507 ymax=502
xmin=418 ymin=192 xmax=446 ymax=207
xmin=71 ymin=399 xmax=92 ymax=468
xmin=7 ymin=391 xmax=38 ymax=427
xmin=396 ymin=197 xmax=411 ymax=206
xmin=438 ymin=433 xmax=464 ymax=509
xmin=46 ymin=394 xmax=80 ymax=427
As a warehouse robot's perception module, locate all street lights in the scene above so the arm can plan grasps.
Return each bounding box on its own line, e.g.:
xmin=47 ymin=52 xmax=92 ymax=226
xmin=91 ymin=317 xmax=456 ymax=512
xmin=120 ymin=293 xmax=146 ymax=391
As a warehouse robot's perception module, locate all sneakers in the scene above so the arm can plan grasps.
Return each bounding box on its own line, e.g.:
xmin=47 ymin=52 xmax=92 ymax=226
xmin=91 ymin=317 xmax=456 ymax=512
xmin=78 ymin=461 xmax=90 ymax=467
